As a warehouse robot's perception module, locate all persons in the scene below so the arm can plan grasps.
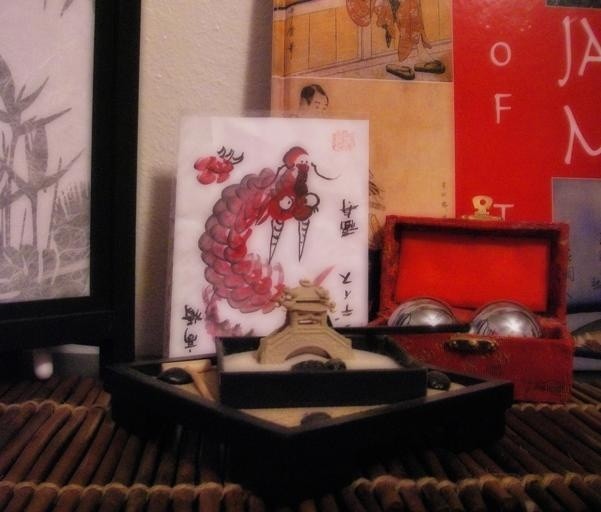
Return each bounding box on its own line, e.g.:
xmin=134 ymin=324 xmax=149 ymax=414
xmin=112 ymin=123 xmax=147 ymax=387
xmin=346 ymin=0 xmax=446 ymax=80
xmin=285 ymin=83 xmax=379 ymax=198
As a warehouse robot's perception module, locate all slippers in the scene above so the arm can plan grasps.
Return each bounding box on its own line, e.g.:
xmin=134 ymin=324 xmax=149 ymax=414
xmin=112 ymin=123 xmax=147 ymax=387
xmin=386 ymin=64 xmax=415 ymax=80
xmin=415 ymin=60 xmax=444 ymax=73
